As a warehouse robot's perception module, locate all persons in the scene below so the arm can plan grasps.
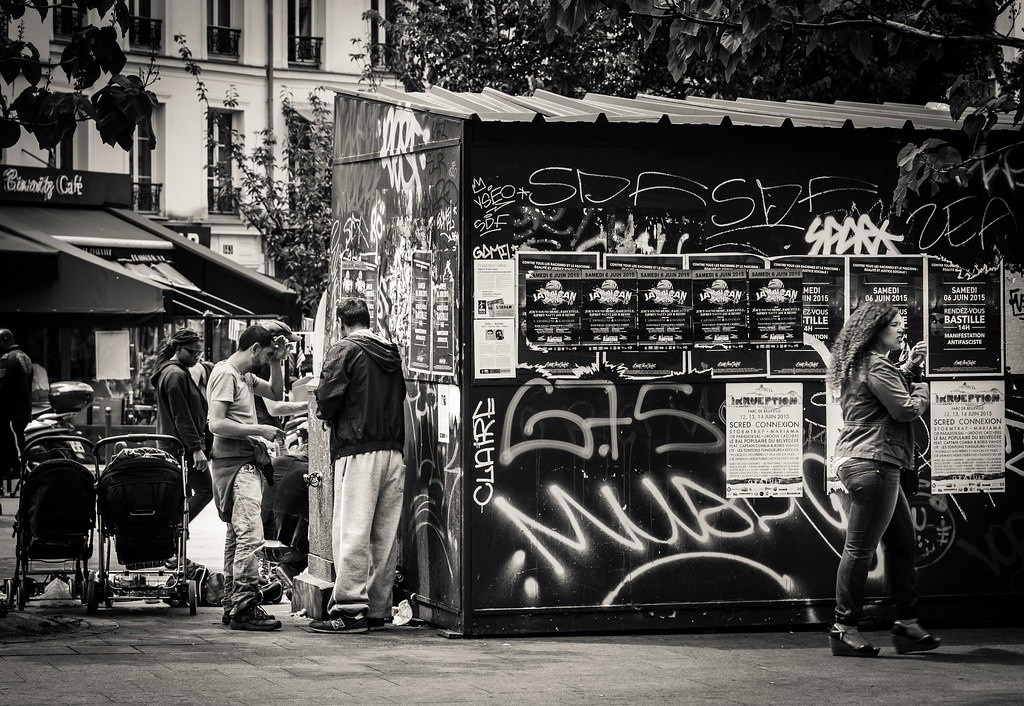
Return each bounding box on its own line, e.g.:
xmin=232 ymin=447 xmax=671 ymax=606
xmin=0 ymin=329 xmax=36 ymax=482
xmin=203 ymin=325 xmax=284 ymax=629
xmin=817 ymin=306 xmax=944 ymax=657
xmin=251 ymin=321 xmax=309 ymax=462
xmin=150 ymin=330 xmax=215 ymax=568
xmin=308 ymin=299 xmax=407 ymax=635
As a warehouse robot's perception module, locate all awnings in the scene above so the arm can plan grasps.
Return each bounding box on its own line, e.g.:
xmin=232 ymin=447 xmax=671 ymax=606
xmin=0 ymin=204 xmax=300 ymax=322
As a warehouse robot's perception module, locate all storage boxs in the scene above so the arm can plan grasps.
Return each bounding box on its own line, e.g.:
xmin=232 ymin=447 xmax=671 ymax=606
xmin=48 ymin=379 xmax=94 ymax=412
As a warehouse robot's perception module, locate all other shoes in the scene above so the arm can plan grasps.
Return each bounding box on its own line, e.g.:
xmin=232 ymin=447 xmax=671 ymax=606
xmin=166 ymin=556 xmax=191 ymax=569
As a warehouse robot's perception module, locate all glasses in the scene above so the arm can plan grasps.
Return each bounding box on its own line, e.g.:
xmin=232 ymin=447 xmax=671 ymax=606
xmin=184 ymin=348 xmax=203 ymax=355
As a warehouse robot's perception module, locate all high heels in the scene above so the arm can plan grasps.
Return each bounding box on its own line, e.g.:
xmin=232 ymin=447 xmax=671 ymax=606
xmin=828 ymin=624 xmax=880 ymax=658
xmin=891 ymin=621 xmax=941 ymax=655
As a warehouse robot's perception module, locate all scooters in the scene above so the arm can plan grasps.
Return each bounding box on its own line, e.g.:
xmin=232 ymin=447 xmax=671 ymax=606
xmin=17 ymin=379 xmax=96 ymax=482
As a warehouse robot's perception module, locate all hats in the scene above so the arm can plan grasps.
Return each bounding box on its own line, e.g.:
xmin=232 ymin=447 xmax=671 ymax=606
xmin=266 ymin=320 xmax=303 ymax=342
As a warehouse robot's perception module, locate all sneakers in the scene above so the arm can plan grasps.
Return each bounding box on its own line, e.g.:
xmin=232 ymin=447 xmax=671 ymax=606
xmin=309 ymin=613 xmax=366 ymax=633
xmin=367 ymin=617 xmax=385 ymax=630
xmin=230 ymin=606 xmax=282 ymax=631
xmin=222 ymin=610 xmax=275 ymax=625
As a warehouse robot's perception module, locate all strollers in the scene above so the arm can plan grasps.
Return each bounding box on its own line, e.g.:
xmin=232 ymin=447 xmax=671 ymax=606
xmin=91 ymin=432 xmax=199 ymax=615
xmin=0 ymin=433 xmax=100 ymax=617
